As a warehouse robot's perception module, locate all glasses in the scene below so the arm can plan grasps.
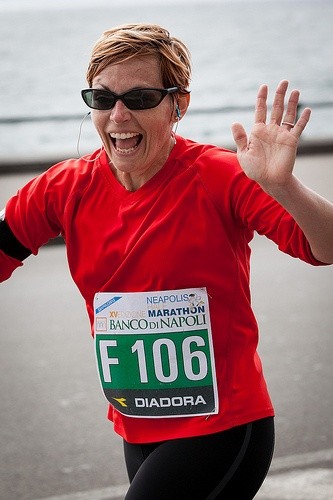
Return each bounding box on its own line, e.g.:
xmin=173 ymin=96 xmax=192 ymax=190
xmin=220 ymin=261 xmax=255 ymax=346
xmin=81 ymin=86 xmax=178 ymax=111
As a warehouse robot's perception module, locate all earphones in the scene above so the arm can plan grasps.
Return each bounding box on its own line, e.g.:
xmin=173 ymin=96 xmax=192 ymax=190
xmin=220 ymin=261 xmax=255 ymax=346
xmin=176 ymin=105 xmax=182 ymax=118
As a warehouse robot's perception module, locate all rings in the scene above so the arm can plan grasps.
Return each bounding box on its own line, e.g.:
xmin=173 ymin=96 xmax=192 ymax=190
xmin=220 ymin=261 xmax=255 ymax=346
xmin=282 ymin=121 xmax=293 ymax=128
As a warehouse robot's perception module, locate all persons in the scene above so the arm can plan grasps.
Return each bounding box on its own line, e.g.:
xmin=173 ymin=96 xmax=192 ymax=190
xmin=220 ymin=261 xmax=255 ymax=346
xmin=0 ymin=21 xmax=333 ymax=500
